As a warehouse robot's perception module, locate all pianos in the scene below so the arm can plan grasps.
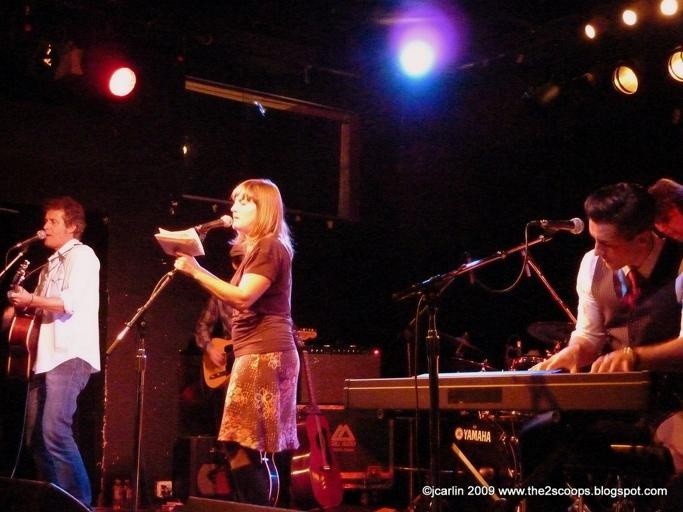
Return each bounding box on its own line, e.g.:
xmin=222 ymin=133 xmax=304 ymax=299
xmin=344 ymin=370 xmax=682 ymax=411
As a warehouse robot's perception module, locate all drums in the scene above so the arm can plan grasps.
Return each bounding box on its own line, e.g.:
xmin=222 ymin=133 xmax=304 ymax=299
xmin=497 ymin=356 xmax=547 ymax=422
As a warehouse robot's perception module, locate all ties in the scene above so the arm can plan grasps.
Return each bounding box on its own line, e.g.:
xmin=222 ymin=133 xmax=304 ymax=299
xmin=620 ymin=271 xmax=641 ymax=307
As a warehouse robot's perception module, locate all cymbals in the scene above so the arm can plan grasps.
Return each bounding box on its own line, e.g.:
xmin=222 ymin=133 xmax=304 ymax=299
xmin=436 ymin=330 xmax=487 ymax=355
xmin=448 ymin=357 xmax=497 ymax=370
xmin=528 ymin=322 xmax=576 ymax=344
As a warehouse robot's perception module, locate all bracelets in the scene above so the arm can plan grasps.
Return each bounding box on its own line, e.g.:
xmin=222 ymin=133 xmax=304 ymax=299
xmin=28 ymin=292 xmax=35 ymax=309
xmin=622 ymin=345 xmax=641 ymax=370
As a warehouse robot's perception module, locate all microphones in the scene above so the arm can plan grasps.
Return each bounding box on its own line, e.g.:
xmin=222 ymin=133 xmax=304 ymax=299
xmin=194 ymin=214 xmax=236 ymax=231
xmin=537 ymin=215 xmax=585 ymax=236
xmin=14 ymin=230 xmax=48 ymax=247
xmin=207 ymin=456 xmax=228 ymax=480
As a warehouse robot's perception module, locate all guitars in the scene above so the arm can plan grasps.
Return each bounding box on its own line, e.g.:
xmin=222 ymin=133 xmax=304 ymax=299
xmin=292 ymin=329 xmax=344 ymax=512
xmin=203 ymin=328 xmax=317 ymax=389
xmin=6 ymin=259 xmax=42 ymax=380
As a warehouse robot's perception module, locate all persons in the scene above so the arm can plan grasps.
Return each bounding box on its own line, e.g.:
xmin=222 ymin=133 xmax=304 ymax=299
xmin=173 ymin=179 xmax=301 ymax=506
xmin=192 ymin=294 xmax=233 ymax=368
xmin=646 ymin=177 xmax=683 ymax=242
xmin=7 ymin=194 xmax=101 ymax=507
xmin=517 ymin=181 xmax=683 ymax=511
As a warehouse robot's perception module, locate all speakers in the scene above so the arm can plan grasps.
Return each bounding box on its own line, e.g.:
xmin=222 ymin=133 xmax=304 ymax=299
xmin=172 ymin=434 xmax=234 ymax=503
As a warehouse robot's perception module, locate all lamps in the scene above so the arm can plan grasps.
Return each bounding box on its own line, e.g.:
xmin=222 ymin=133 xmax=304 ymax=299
xmin=609 ymin=58 xmax=641 ymax=97
xmin=665 ymin=42 xmax=683 ymax=86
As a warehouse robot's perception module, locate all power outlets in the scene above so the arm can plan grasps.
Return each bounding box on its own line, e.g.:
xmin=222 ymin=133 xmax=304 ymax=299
xmin=155 ymin=479 xmax=172 ymax=499
